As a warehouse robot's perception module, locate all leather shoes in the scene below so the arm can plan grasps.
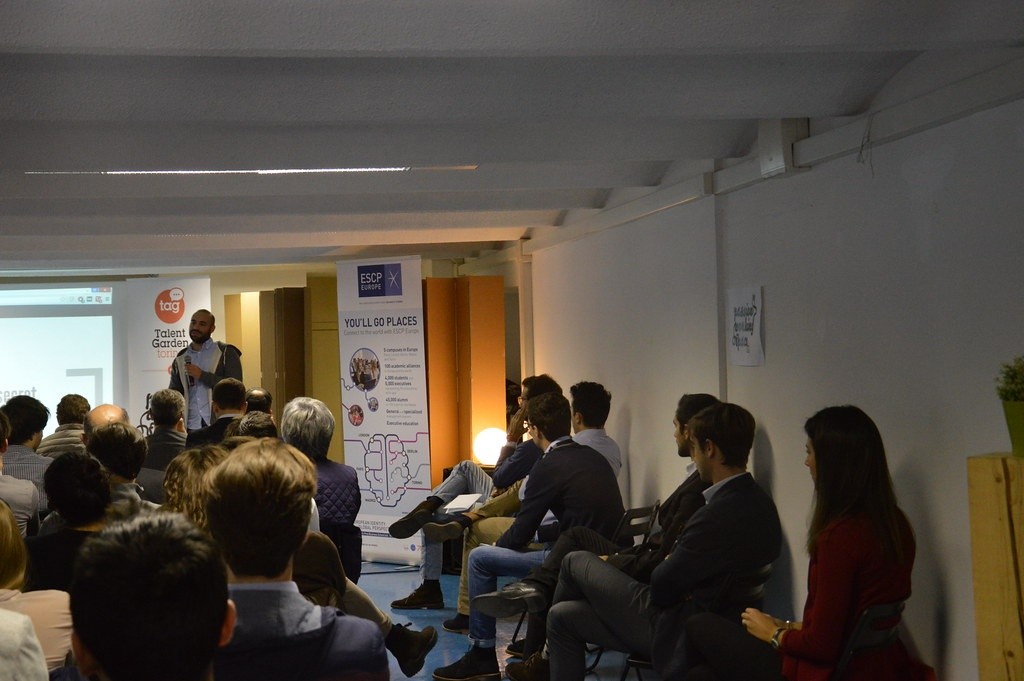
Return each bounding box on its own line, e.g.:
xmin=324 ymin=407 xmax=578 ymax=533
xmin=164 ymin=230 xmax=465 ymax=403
xmin=388 ymin=501 xmax=434 ymax=539
xmin=472 ymin=578 xmax=550 ymax=617
xmin=391 ymin=584 xmax=444 ymax=609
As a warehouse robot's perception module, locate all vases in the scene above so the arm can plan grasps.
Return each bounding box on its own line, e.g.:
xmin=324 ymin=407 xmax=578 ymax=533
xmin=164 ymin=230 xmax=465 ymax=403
xmin=1002 ymin=402 xmax=1024 ymax=456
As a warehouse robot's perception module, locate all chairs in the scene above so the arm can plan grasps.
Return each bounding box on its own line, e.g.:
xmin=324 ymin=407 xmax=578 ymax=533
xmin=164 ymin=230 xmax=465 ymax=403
xmin=511 ymin=498 xmax=906 ymax=681
xmin=322 ymin=520 xmax=362 ymax=584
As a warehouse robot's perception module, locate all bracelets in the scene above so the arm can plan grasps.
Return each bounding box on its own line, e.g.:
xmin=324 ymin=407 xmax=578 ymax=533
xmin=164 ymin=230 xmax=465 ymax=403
xmin=785 ymin=620 xmax=792 ymax=630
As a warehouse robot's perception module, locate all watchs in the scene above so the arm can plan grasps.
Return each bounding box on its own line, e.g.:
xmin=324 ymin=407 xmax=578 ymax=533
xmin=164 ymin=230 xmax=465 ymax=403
xmin=771 ymin=627 xmax=785 ymax=648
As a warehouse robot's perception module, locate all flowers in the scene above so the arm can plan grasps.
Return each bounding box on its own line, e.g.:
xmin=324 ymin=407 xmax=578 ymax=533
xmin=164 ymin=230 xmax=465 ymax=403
xmin=994 ymin=354 xmax=1024 ymax=403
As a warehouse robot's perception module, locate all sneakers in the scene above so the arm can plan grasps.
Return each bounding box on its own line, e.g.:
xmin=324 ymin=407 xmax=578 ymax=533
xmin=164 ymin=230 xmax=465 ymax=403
xmin=385 ymin=622 xmax=438 ymax=677
xmin=423 ymin=513 xmax=470 ymax=543
xmin=442 ymin=614 xmax=470 ymax=634
xmin=505 ymin=652 xmax=549 ymax=681
xmin=506 ymin=638 xmax=526 ymax=657
xmin=432 ymin=645 xmax=501 ymax=681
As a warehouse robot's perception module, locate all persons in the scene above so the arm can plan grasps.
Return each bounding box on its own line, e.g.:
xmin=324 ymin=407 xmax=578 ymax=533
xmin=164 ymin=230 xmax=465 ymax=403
xmin=169 ymin=309 xmax=244 ymax=431
xmin=349 ymin=358 xmax=379 ymax=426
xmin=0 ymin=376 xmax=935 ymax=681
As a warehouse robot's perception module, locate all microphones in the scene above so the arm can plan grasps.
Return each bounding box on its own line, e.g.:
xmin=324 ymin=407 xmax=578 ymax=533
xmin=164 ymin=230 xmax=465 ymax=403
xmin=184 ymin=355 xmax=194 ymax=386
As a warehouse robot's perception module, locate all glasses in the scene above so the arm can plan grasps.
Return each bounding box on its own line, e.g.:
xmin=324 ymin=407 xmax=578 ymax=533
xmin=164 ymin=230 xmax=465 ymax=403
xmin=523 ymin=420 xmax=534 ymax=428
xmin=518 ymin=397 xmax=528 ymax=405
